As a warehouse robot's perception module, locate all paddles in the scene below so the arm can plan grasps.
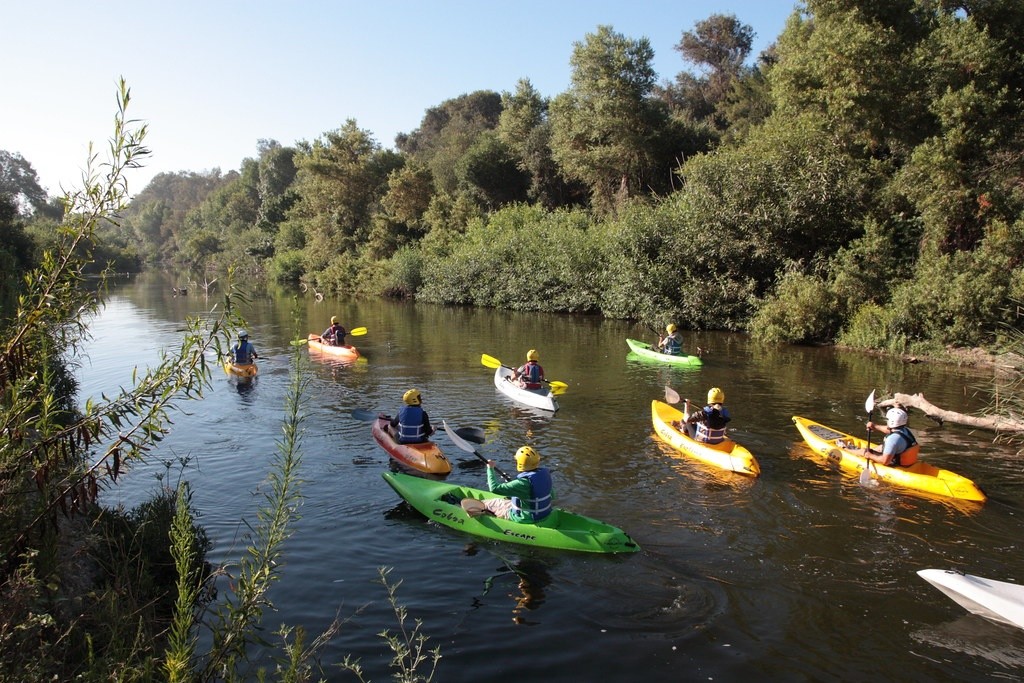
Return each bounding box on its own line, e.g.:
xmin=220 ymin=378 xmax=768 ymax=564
xmin=481 ymin=354 xmax=568 ymax=388
xmin=860 ymin=389 xmax=876 ymax=481
xmin=443 ymin=420 xmax=512 ymax=482
xmin=204 ymin=351 xmax=272 ymax=360
xmin=290 ymin=326 xmax=367 ymax=346
xmin=645 ymin=322 xmax=684 ymax=353
xmin=665 ymin=386 xmax=703 ymax=411
xmin=351 ymin=408 xmax=485 ymax=444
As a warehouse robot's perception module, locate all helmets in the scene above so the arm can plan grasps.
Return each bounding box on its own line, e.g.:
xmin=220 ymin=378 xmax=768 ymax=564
xmin=516 ymin=446 xmax=540 ymax=473
xmin=885 ymin=407 xmax=908 ymax=429
xmin=331 ymin=315 xmax=339 ymax=325
xmin=707 ymin=387 xmax=724 ymax=404
xmin=526 ymin=349 xmax=539 ymax=362
xmin=402 ymin=389 xmax=421 ymax=406
xmin=666 ymin=324 xmax=676 ymax=335
xmin=237 ymin=331 xmax=248 ymax=341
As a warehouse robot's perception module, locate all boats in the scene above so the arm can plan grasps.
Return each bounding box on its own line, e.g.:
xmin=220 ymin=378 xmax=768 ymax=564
xmin=494 ymin=365 xmax=559 ymax=412
xmin=224 ymin=355 xmax=258 ymax=377
xmin=370 ymin=413 xmax=451 ymax=474
xmin=173 ymin=287 xmax=187 ymax=295
xmin=626 ymin=337 xmax=704 ymax=365
xmin=917 ymin=568 xmax=1024 ymax=631
xmin=309 ymin=334 xmax=361 ymax=360
xmin=651 ymin=401 xmax=762 ymax=477
xmin=793 ymin=414 xmax=986 ymax=501
xmin=383 ymin=473 xmax=642 ymax=553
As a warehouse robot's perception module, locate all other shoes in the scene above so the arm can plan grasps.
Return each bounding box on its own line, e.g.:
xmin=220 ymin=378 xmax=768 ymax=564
xmin=681 ymin=419 xmax=686 ymax=430
xmin=671 ymin=420 xmax=680 ymax=431
xmin=848 ymin=441 xmax=858 ymax=450
xmin=836 ymin=438 xmax=847 ymax=449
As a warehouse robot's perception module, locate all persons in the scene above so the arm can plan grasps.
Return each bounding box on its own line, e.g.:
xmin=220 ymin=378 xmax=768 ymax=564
xmin=836 ymin=407 xmax=920 ymax=466
xmin=650 ymin=323 xmax=685 ymax=355
xmin=511 ymin=349 xmax=544 ymax=390
xmin=671 ymin=387 xmax=731 ymax=445
xmin=449 ymin=445 xmax=553 ymax=523
xmin=388 ymin=389 xmax=437 ymax=444
xmin=320 ymin=316 xmax=346 ymax=346
xmin=226 ymin=330 xmax=258 ymax=365
